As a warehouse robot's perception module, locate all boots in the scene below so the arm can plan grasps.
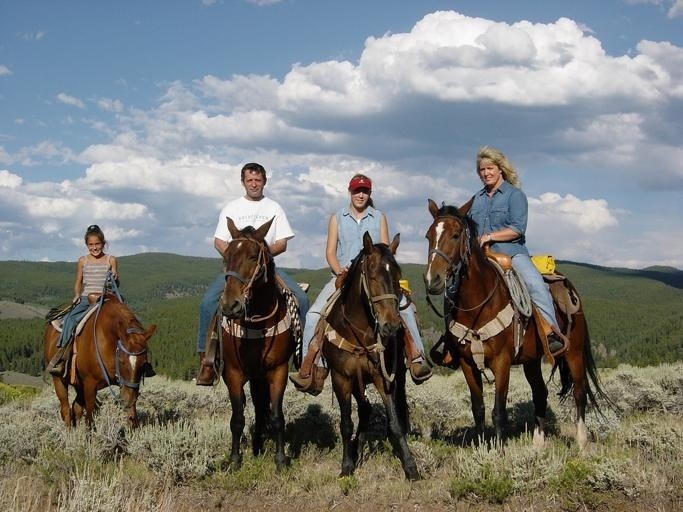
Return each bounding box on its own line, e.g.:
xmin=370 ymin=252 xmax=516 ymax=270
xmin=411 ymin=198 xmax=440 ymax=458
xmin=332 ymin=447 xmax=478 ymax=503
xmin=289 ymin=367 xmax=329 ymax=396
xmin=196 ymin=367 xmax=219 ymax=385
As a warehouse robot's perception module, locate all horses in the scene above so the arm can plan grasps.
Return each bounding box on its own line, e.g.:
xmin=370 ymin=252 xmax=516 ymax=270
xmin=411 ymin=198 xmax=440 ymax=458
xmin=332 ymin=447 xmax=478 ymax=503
xmin=41 ymin=294 xmax=157 ymax=436
xmin=214 ymin=214 xmax=301 ymax=476
xmin=322 ymin=231 xmax=430 ymax=491
xmin=422 ymin=194 xmax=624 ymax=458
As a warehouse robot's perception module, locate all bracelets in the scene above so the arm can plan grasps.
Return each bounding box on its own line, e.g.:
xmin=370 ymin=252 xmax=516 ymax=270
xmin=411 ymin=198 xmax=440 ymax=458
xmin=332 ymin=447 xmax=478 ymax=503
xmin=486 ymin=233 xmax=493 ymax=241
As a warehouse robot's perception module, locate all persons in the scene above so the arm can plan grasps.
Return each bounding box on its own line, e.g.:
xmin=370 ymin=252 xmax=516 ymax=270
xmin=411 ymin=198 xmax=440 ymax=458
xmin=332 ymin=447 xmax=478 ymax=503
xmin=436 ymin=142 xmax=571 ymax=371
xmin=193 ymin=160 xmax=310 ymax=388
xmin=43 ymin=225 xmax=120 ymax=378
xmin=286 ymin=172 xmax=435 ymax=397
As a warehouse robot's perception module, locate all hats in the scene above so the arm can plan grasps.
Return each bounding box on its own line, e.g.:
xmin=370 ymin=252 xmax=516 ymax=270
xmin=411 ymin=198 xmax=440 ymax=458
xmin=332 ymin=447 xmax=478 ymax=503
xmin=348 ymin=174 xmax=373 ymax=192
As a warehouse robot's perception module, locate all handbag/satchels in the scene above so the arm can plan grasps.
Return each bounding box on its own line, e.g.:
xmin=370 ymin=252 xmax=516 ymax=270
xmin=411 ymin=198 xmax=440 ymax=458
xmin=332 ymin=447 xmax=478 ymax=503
xmin=531 ymin=255 xmax=556 ymax=274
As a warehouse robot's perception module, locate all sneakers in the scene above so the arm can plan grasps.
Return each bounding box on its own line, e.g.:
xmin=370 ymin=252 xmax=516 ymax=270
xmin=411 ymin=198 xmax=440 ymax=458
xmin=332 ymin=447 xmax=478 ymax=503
xmin=549 ymin=336 xmax=564 ymax=354
xmin=49 ymin=360 xmax=64 ymax=376
xmin=409 ymin=363 xmax=430 ymax=385
